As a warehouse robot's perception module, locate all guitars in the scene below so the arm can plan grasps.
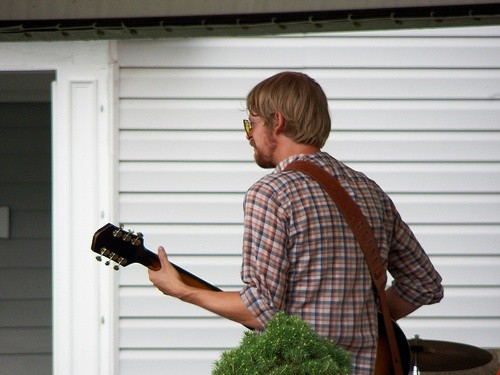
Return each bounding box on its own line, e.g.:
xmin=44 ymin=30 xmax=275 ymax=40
xmin=90 ymin=223 xmax=412 ymax=375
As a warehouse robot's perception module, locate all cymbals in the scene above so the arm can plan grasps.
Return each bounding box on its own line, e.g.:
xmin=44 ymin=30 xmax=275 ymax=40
xmin=409 ymin=335 xmax=492 ymax=373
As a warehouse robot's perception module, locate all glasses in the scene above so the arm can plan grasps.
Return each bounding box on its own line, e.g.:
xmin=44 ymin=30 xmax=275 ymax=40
xmin=243 ymin=117 xmax=266 ymax=136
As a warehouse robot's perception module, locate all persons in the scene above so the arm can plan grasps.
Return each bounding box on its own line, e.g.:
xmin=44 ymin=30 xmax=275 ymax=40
xmin=149 ymin=72 xmax=442 ymax=375
